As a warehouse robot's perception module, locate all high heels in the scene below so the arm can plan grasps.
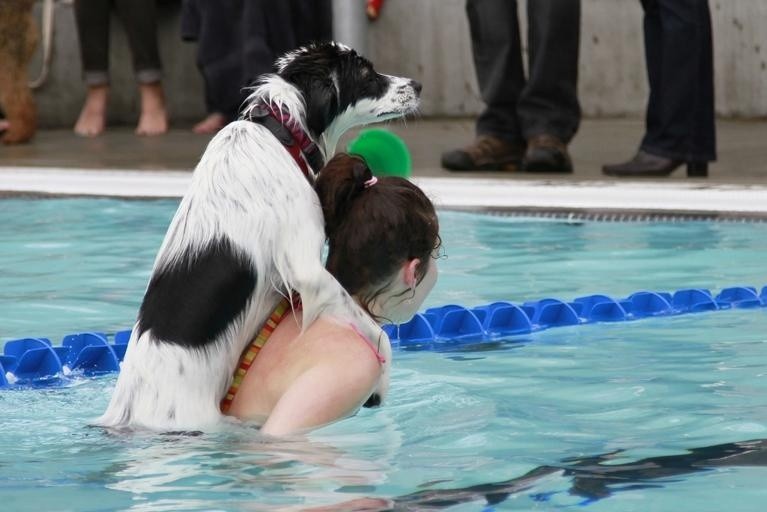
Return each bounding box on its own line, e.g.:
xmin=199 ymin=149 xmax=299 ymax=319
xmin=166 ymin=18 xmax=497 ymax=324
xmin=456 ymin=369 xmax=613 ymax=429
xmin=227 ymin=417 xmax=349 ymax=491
xmin=602 ymin=148 xmax=710 ymax=177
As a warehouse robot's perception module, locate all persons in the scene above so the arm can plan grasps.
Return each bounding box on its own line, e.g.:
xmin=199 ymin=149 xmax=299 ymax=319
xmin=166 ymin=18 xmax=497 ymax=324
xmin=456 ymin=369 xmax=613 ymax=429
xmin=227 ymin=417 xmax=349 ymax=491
xmin=213 ymin=150 xmax=445 ymax=486
xmin=598 ymin=2 xmax=717 ymax=178
xmin=67 ymin=0 xmax=171 ymax=139
xmin=181 ymin=0 xmax=332 ymax=137
xmin=439 ymin=1 xmax=582 ymax=175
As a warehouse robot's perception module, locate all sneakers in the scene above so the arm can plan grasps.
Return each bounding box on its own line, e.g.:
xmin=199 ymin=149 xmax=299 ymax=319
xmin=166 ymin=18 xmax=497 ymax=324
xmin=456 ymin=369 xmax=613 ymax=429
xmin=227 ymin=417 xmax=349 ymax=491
xmin=440 ymin=132 xmax=522 ymax=171
xmin=521 ymin=133 xmax=575 ymax=173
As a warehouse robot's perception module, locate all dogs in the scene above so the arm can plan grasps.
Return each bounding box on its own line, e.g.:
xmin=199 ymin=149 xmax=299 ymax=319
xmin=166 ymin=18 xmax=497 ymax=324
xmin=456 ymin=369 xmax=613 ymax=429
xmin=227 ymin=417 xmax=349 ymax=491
xmin=85 ymin=41 xmax=424 ymax=436
